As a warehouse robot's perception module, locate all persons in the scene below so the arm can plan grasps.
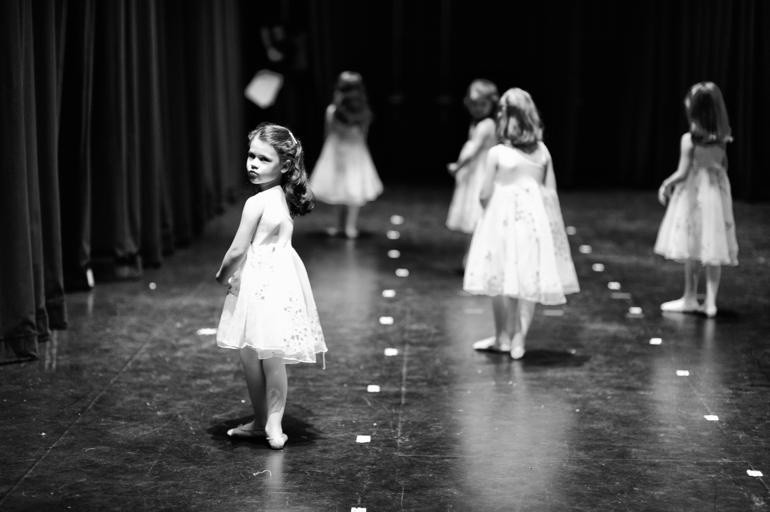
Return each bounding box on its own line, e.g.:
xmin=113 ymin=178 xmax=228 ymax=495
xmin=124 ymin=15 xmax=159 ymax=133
xmin=214 ymin=121 xmax=330 ymax=451
xmin=459 ymin=85 xmax=583 ymax=364
xmin=655 ymin=77 xmax=741 ymax=322
xmin=304 ymin=70 xmax=385 ymax=240
xmin=441 ymin=76 xmax=502 ymax=275
